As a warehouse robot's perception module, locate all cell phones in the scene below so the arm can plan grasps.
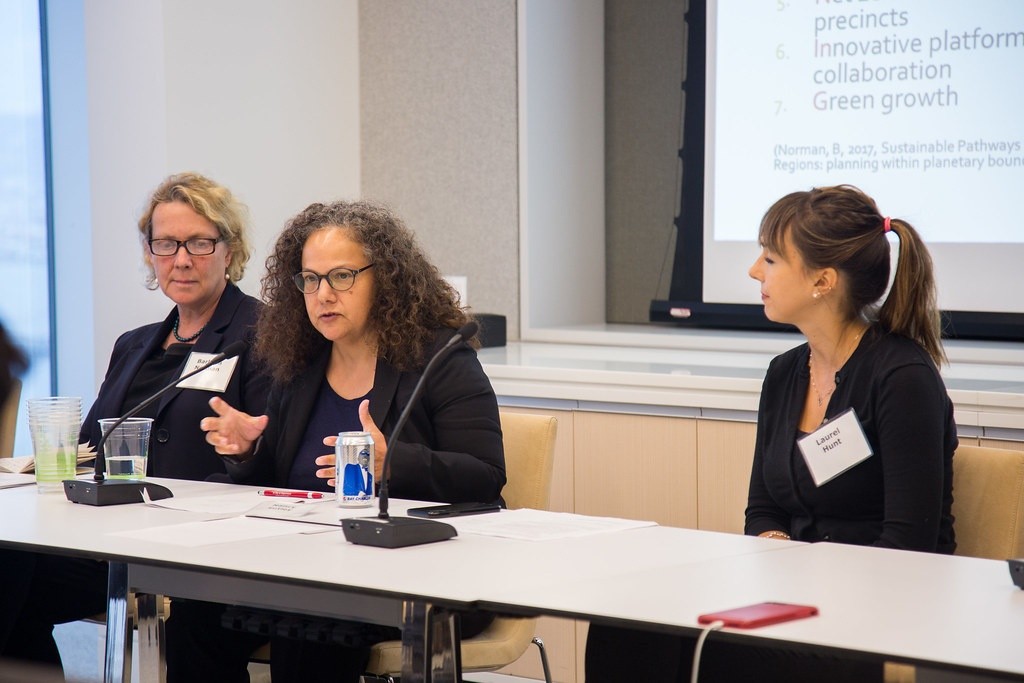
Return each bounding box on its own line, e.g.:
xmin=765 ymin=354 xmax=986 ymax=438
xmin=697 ymin=600 xmax=817 ymax=630
xmin=407 ymin=502 xmax=502 ymax=518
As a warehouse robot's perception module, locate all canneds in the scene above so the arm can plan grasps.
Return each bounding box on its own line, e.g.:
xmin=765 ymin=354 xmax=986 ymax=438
xmin=334 ymin=431 xmax=376 ymax=509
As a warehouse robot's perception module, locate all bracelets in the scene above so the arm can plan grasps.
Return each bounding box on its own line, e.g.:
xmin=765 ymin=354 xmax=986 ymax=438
xmin=765 ymin=532 xmax=790 ymax=539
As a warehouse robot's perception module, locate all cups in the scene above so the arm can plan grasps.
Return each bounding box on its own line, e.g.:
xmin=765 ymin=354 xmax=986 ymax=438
xmin=28 ymin=397 xmax=85 ymax=492
xmin=98 ymin=418 xmax=154 ymax=479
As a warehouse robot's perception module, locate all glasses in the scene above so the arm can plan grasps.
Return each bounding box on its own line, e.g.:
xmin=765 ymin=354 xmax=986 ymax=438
xmin=149 ymin=230 xmax=225 ymax=256
xmin=293 ymin=263 xmax=374 ymax=294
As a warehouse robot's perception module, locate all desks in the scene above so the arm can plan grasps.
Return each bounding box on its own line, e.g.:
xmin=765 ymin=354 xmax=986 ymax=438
xmin=0 ymin=467 xmax=1024 ymax=683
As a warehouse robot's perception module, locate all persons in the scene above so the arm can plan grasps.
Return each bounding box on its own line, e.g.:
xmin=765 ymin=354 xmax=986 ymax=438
xmin=344 ymin=449 xmax=373 ymax=497
xmin=0 ymin=172 xmax=276 ymax=683
xmin=585 ymin=187 xmax=959 ymax=683
xmin=158 ymin=197 xmax=507 ymax=683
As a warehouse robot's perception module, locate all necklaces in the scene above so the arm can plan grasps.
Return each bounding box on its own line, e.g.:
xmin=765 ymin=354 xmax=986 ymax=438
xmin=173 ymin=316 xmax=211 ymax=343
xmin=808 ymin=320 xmax=868 ymax=408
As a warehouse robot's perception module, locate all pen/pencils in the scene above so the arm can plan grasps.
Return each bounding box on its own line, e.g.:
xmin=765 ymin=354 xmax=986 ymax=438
xmin=258 ymin=489 xmax=325 ymax=499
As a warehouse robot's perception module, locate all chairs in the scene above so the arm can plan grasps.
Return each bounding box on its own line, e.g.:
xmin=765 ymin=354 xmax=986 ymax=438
xmin=246 ymin=413 xmax=558 ymax=683
xmin=884 ymin=446 xmax=1023 ymax=683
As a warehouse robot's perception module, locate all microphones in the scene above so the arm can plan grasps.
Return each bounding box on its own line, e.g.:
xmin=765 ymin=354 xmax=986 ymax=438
xmin=339 ymin=321 xmax=478 ymax=548
xmin=62 ymin=340 xmax=246 ymax=506
